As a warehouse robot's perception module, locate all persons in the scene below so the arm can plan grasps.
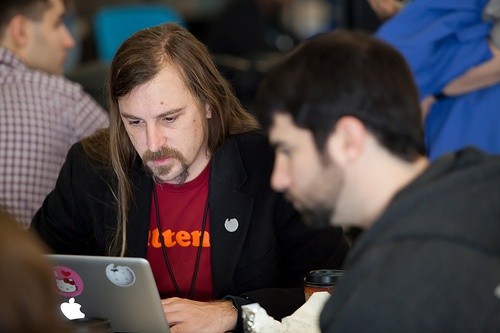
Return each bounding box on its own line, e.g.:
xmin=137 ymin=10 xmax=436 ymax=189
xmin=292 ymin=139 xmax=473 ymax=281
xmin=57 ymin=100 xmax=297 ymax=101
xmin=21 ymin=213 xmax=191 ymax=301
xmin=240 ymin=24 xmax=500 ymax=333
xmin=0 ymin=207 xmax=76 ymax=333
xmin=29 ymin=24 xmax=350 ymax=333
xmin=0 ymin=0 xmax=111 ymax=231
xmin=366 ymin=0 xmax=500 ymax=158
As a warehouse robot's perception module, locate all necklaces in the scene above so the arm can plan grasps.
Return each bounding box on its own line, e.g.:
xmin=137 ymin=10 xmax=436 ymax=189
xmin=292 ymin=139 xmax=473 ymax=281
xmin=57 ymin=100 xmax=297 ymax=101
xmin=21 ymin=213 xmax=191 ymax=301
xmin=151 ymin=175 xmax=209 ymax=300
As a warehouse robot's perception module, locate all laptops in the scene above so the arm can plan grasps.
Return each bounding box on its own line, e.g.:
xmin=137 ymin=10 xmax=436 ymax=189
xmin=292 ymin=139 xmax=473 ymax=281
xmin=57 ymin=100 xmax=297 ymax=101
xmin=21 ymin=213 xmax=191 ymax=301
xmin=41 ymin=253 xmax=171 ymax=333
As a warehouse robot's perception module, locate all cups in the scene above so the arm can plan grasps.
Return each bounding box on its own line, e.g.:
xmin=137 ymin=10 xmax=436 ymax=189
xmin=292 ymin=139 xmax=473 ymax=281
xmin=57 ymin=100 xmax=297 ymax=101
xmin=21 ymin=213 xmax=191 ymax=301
xmin=303 ymin=269 xmax=342 ymax=301
